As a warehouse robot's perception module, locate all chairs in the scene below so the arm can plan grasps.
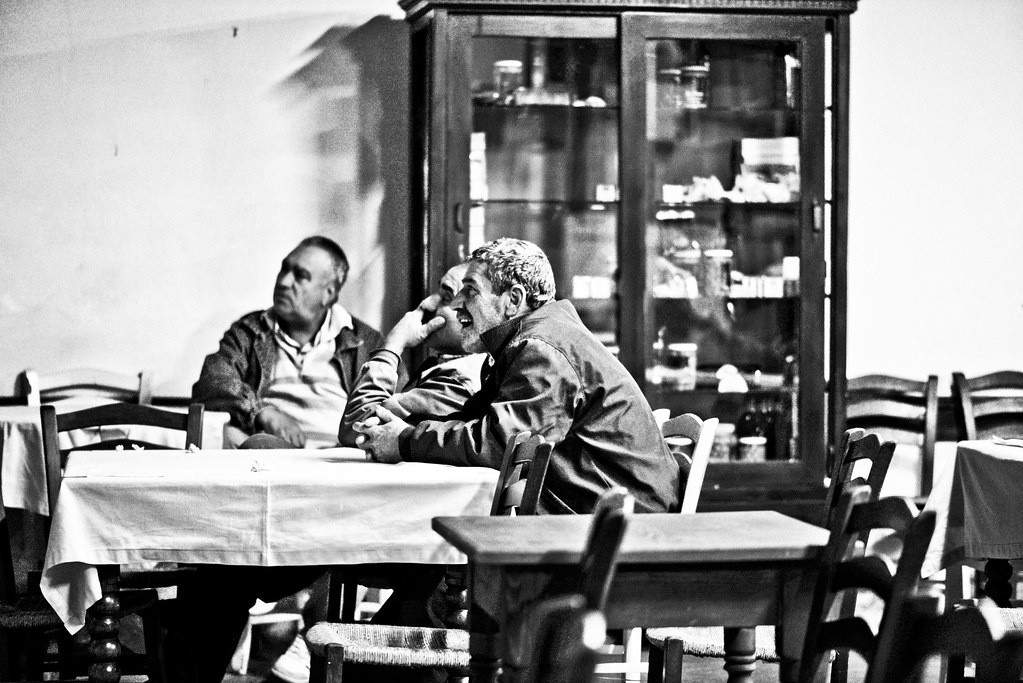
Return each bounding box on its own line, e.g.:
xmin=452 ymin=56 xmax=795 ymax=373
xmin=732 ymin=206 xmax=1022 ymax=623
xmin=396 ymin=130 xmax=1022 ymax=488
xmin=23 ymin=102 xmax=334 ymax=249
xmin=14 ymin=370 xmax=1023 ymax=683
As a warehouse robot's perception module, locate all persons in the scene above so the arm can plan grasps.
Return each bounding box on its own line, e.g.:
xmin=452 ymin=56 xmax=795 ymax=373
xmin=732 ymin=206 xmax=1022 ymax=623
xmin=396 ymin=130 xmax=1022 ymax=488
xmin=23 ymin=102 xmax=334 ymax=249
xmin=162 ymin=235 xmax=425 ymax=683
xmin=337 ymin=264 xmax=487 ymax=448
xmin=359 ymin=236 xmax=681 ymax=516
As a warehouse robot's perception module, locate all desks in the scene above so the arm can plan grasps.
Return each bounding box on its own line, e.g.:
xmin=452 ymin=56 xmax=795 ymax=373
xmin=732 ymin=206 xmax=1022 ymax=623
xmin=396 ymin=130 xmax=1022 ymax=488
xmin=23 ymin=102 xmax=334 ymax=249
xmin=0 ymin=399 xmax=230 ymax=602
xmin=40 ymin=445 xmax=503 ymax=683
xmin=937 ymin=437 xmax=1023 ymax=611
xmin=431 ymin=510 xmax=863 ymax=683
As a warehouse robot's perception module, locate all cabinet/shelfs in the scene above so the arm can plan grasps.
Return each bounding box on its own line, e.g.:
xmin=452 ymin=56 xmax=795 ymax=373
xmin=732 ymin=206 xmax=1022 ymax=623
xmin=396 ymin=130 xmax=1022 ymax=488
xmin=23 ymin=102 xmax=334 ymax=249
xmin=398 ymin=1 xmax=859 ymax=520
